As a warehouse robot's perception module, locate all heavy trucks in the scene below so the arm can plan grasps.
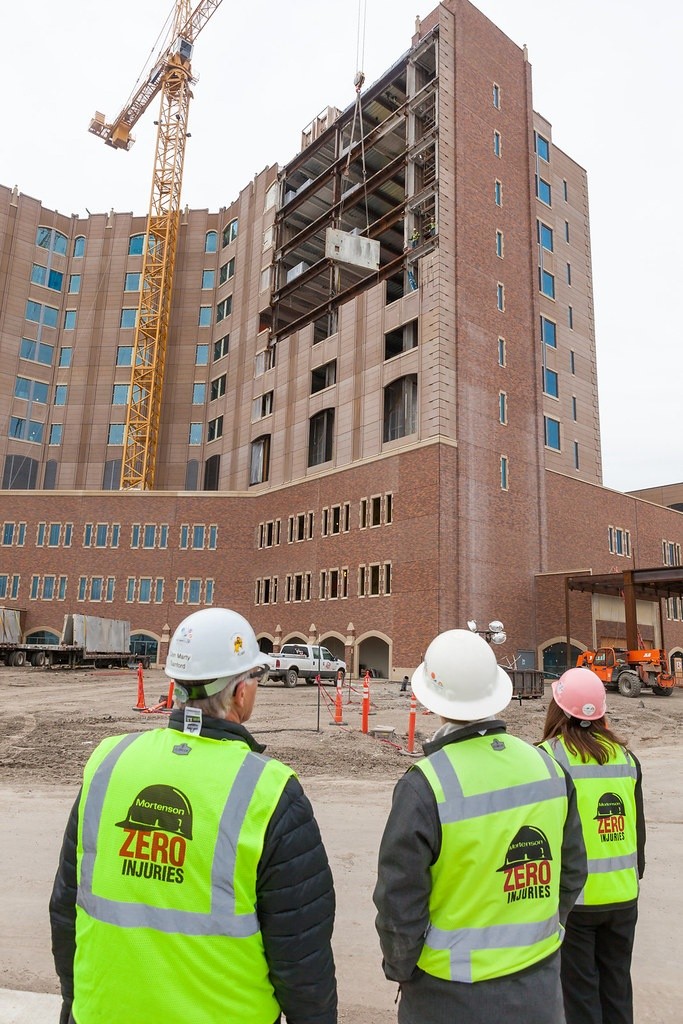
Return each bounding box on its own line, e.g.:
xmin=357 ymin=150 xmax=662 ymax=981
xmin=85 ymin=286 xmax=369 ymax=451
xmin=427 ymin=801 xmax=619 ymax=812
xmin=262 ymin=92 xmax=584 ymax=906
xmin=0 ymin=606 xmax=151 ymax=669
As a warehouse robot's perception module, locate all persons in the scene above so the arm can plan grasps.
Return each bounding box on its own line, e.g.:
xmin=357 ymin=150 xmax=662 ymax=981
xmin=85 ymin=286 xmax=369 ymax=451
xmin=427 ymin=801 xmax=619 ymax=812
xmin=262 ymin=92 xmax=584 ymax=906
xmin=429 ymin=217 xmax=435 ymax=236
xmin=408 ymin=228 xmax=420 ymax=248
xmin=533 ymin=667 xmax=647 ymax=1024
xmin=48 ymin=607 xmax=339 ymax=1024
xmin=372 ymin=628 xmax=588 ymax=1024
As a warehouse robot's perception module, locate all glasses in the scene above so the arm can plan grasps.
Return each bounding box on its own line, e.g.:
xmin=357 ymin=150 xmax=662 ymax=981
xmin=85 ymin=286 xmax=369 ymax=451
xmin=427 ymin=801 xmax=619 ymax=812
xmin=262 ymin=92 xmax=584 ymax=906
xmin=233 ymin=664 xmax=271 ymax=697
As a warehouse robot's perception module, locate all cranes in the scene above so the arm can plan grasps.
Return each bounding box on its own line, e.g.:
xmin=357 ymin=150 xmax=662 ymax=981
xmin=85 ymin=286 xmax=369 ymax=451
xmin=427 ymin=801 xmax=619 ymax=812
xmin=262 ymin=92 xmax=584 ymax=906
xmin=87 ymin=0 xmax=224 ymax=490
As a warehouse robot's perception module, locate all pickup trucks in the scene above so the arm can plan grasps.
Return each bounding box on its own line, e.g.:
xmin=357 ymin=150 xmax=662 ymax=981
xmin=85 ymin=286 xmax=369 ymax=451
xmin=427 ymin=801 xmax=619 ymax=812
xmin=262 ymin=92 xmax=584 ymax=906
xmin=261 ymin=641 xmax=348 ymax=689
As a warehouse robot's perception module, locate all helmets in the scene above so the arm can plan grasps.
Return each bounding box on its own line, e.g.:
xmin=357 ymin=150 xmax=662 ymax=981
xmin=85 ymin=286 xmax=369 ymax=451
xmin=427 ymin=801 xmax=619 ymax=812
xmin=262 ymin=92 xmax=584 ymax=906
xmin=551 ymin=668 xmax=606 ymax=720
xmin=411 ymin=629 xmax=513 ymax=721
xmin=164 ymin=608 xmax=271 ymax=680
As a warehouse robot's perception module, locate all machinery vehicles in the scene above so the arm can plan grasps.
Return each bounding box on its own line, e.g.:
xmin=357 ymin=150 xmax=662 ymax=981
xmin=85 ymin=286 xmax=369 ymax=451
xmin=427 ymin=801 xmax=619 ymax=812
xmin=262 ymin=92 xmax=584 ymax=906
xmin=574 ymin=645 xmax=676 ymax=698
